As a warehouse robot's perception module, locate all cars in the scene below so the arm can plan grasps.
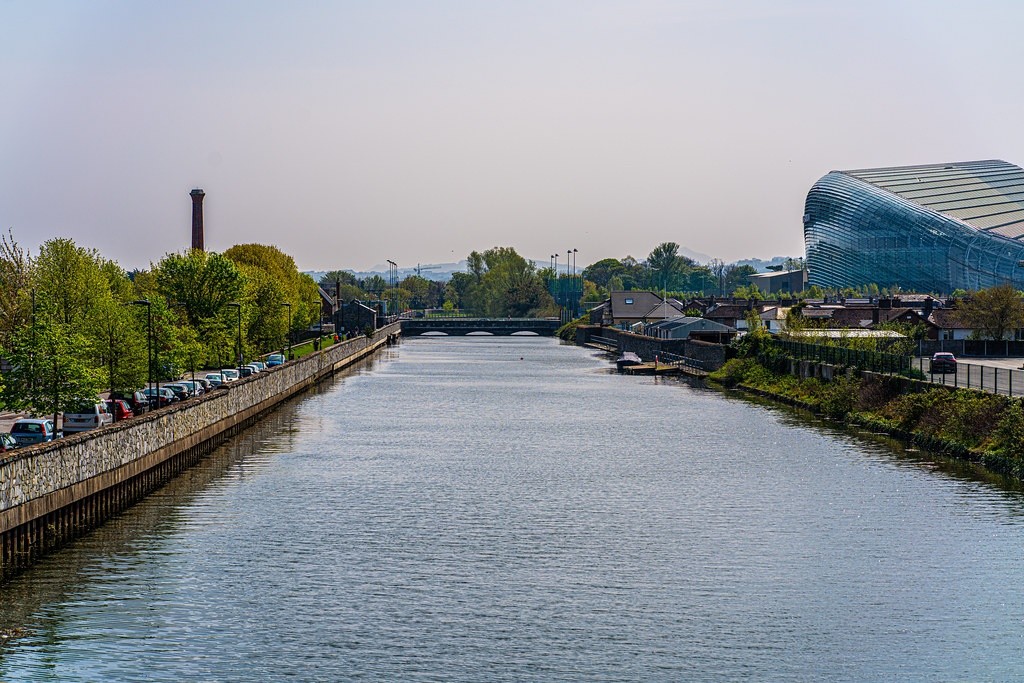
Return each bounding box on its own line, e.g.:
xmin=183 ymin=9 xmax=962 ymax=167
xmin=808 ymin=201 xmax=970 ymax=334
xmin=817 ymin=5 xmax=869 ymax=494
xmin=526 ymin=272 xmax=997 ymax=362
xmin=178 ymin=381 xmax=205 ymax=398
xmin=143 ymin=387 xmax=180 ymax=410
xmin=108 ymin=389 xmax=152 ymax=417
xmin=206 ymin=373 xmax=227 ymax=389
xmin=219 ymin=354 xmax=285 ymax=383
xmin=62 ymin=398 xmax=113 ymax=438
xmin=929 ymin=352 xmax=957 ymax=373
xmin=157 ymin=364 xmax=184 ymax=382
xmin=105 ymin=398 xmax=134 ymax=423
xmin=9 ymin=419 xmax=64 ymax=449
xmin=188 ymin=378 xmax=215 ymax=393
xmin=163 ymin=384 xmax=192 ymax=400
